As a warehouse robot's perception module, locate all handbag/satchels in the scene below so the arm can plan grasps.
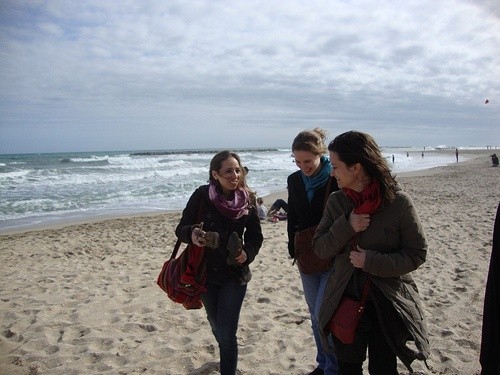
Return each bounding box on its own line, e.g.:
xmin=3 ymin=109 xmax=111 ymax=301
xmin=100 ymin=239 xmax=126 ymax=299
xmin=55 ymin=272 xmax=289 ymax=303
xmin=157 ymin=242 xmax=207 ymax=310
xmin=292 ymin=224 xmax=333 ymax=273
xmin=326 ymin=295 xmax=365 ymax=344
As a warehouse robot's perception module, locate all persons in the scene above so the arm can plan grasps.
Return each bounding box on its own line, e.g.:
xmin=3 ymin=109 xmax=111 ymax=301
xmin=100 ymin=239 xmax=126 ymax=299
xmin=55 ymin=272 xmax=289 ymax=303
xmin=287 ymin=127 xmax=343 ymax=375
xmin=311 ymin=130 xmax=428 ymax=375
xmin=256 ymin=198 xmax=288 ymax=220
xmin=175 ymin=151 xmax=264 ymax=375
